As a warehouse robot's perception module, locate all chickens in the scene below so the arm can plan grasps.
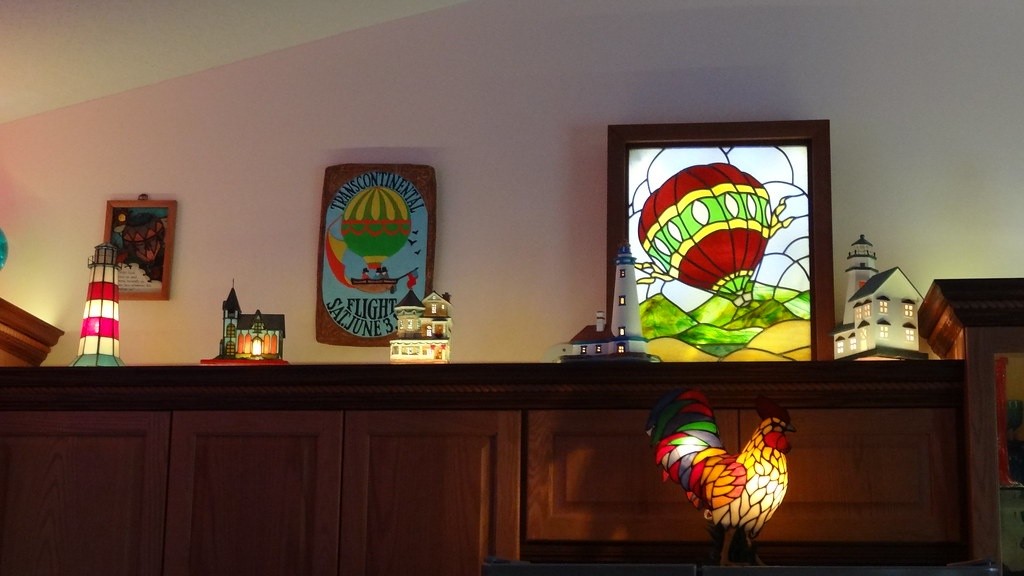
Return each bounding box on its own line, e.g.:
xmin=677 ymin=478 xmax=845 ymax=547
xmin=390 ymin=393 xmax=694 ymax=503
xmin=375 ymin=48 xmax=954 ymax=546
xmin=642 ymin=382 xmax=798 ymax=565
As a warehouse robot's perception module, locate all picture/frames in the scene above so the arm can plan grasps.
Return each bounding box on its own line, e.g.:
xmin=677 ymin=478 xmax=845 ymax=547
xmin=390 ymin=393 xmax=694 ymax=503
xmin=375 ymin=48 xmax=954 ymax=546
xmin=103 ymin=200 xmax=177 ymax=300
xmin=606 ymin=118 xmax=834 ymax=361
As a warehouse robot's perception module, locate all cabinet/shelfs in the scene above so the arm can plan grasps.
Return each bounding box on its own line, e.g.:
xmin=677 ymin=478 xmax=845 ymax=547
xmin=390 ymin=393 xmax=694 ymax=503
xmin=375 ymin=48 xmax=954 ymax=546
xmin=0 ymin=361 xmax=967 ymax=576
